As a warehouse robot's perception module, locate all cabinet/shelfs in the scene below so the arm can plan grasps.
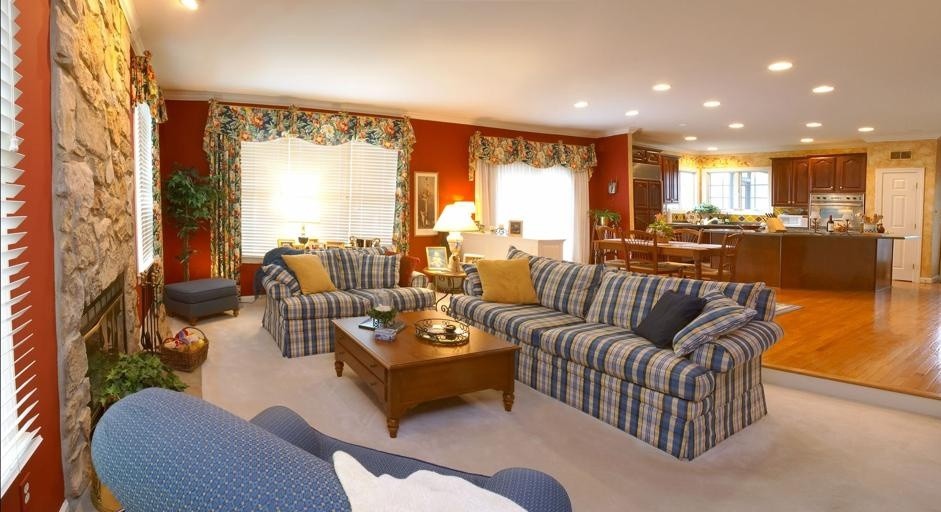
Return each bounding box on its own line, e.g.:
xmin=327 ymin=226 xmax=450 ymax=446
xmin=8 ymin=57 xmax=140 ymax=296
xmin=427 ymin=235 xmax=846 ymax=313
xmin=770 ymin=157 xmax=809 ymax=207
xmin=632 ymin=143 xmax=661 ymax=231
xmin=661 ymin=153 xmax=682 ymax=204
xmin=808 ymin=151 xmax=867 ymax=194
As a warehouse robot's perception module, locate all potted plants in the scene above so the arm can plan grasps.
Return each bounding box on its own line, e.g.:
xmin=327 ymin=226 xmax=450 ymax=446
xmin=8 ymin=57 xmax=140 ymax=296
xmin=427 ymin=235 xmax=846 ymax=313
xmin=594 ymin=208 xmax=618 ymax=227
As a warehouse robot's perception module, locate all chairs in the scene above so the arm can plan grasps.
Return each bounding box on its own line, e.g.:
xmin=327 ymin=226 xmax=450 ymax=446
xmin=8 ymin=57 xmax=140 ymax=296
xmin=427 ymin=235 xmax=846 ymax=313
xmin=595 ymin=224 xmax=743 ymax=284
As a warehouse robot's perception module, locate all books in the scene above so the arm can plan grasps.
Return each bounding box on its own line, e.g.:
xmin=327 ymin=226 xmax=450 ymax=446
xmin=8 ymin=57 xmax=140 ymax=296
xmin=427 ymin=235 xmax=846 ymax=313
xmin=359 ymin=316 xmax=406 ymax=333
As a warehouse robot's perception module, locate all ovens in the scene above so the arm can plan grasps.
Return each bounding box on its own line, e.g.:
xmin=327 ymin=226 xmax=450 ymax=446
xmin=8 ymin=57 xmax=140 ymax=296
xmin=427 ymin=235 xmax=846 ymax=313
xmin=810 ymin=194 xmax=864 ymax=226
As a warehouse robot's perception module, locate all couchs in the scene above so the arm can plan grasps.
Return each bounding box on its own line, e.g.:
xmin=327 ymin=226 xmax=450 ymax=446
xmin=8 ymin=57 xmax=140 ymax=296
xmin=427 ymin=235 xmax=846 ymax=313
xmin=258 ymin=247 xmax=436 ymax=358
xmin=447 ymin=245 xmax=784 ymax=463
xmin=92 ymin=386 xmax=571 ymax=511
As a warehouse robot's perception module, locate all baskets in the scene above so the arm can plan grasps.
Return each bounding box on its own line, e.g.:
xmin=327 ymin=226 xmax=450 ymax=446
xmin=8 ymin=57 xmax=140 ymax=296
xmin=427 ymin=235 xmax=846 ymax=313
xmin=159 ymin=326 xmax=209 ymax=372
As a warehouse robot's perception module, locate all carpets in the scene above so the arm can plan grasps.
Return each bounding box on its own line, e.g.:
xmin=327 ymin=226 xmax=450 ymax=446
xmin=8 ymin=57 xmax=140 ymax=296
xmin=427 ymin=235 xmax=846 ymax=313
xmin=775 ymin=302 xmax=803 ymax=316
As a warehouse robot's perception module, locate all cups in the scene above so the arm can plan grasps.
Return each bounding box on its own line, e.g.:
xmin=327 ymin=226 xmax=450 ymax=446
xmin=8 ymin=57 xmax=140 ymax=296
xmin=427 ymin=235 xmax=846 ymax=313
xmin=297 ymin=236 xmax=323 ymax=250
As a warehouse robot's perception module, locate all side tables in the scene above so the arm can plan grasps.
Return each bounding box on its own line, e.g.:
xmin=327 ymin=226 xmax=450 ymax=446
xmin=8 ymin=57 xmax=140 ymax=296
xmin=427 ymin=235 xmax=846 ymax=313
xmin=421 ymin=260 xmax=464 ymax=315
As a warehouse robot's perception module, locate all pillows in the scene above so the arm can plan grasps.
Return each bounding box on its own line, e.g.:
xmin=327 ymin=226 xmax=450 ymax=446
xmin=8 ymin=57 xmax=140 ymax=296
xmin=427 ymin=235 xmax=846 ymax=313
xmin=279 ymin=253 xmax=338 ymax=297
xmin=355 ymin=251 xmax=401 ymax=289
xmin=358 ymin=247 xmax=388 ymax=255
xmin=305 ymin=246 xmax=359 ymax=290
xmin=633 ymin=289 xmax=706 ymax=349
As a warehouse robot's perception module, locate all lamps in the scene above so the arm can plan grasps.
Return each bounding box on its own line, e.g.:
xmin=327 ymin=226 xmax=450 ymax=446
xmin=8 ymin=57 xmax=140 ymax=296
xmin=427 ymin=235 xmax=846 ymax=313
xmin=433 ymin=205 xmax=480 ymax=272
xmin=285 ymin=201 xmax=320 ymax=244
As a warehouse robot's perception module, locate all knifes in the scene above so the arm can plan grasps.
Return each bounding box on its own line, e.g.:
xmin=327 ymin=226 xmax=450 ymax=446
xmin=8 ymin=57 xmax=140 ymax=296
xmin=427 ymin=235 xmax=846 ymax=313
xmin=762 ymin=213 xmax=777 ymax=222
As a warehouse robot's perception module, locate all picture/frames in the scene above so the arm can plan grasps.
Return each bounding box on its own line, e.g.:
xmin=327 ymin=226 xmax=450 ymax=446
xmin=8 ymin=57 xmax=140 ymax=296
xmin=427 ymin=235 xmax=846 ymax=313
xmin=414 ymin=170 xmax=440 ymax=238
xmin=508 ymin=220 xmax=523 ymax=237
xmin=426 ymin=246 xmax=448 ymax=271
xmin=278 ymin=239 xmax=296 ymax=248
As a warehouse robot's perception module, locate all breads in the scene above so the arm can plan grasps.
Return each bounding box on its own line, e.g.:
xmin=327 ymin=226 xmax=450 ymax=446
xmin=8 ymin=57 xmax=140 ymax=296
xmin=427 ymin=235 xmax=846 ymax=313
xmin=863 ymin=214 xmax=883 ymax=224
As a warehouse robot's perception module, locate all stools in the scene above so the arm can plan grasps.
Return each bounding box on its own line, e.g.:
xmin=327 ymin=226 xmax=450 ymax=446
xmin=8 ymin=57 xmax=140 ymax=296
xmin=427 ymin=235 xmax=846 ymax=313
xmin=164 ymin=277 xmax=239 ymax=326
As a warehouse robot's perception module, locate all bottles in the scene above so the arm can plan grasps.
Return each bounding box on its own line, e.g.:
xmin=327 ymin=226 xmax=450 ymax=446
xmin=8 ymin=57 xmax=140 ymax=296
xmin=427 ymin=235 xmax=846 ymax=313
xmin=667 ymin=208 xmax=672 ymax=224
xmin=826 ymin=215 xmax=835 ymax=232
xmin=878 ymin=222 xmax=885 ymax=233
xmin=356 ymin=239 xmax=372 ymax=247
xmin=859 ymin=222 xmax=864 ymax=233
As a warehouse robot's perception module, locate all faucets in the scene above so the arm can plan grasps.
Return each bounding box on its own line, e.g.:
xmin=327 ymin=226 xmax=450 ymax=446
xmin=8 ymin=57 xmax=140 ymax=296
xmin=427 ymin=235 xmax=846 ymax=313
xmin=736 ymin=224 xmax=743 ymax=230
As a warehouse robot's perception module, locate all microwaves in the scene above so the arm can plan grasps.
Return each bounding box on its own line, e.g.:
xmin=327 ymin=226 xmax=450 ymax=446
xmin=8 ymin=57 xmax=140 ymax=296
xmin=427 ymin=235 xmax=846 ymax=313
xmin=778 ymin=215 xmax=809 ymax=228
